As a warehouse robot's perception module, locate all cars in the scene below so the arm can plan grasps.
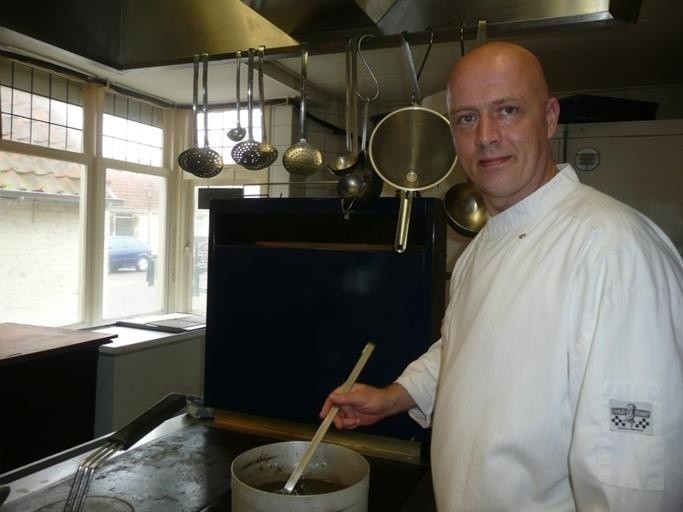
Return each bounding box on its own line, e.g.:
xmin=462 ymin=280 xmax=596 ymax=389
xmin=106 ymin=235 xmax=152 ymax=274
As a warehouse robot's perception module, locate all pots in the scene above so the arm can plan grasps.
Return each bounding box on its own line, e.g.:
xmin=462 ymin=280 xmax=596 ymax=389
xmin=231 ymin=441 xmax=371 ymax=512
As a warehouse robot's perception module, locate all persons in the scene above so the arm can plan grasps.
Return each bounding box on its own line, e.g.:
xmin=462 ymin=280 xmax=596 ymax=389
xmin=318 ymin=40 xmax=682 ymax=511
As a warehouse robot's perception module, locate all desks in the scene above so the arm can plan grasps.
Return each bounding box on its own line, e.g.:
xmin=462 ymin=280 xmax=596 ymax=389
xmin=0 ymin=318 xmax=119 ymax=476
xmin=96 ymin=313 xmax=206 ymax=438
